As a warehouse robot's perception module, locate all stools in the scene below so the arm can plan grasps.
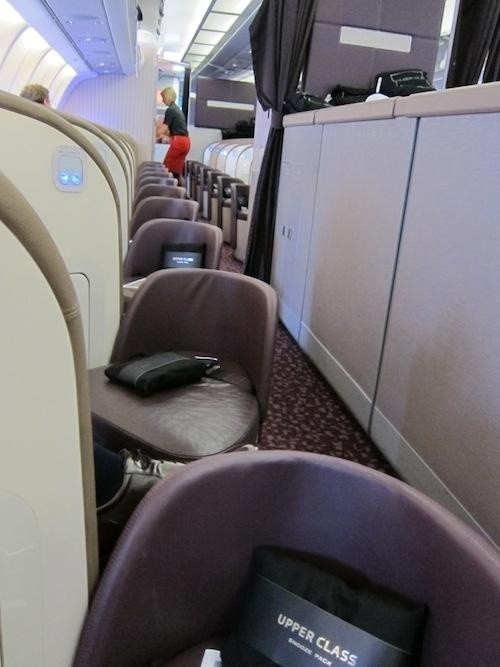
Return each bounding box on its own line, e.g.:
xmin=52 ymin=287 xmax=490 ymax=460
xmin=134 ymin=159 xmax=189 ymax=207
xmin=122 ymin=215 xmax=226 ymax=308
xmin=72 ymin=446 xmax=498 ymax=665
xmin=128 ymin=194 xmax=200 ymax=245
xmin=86 ymin=268 xmax=285 ymax=514
xmin=185 ymin=161 xmax=255 ymax=264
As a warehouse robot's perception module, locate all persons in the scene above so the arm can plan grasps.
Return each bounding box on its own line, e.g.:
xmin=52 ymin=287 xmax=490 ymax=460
xmin=21 ymin=84 xmax=50 ymax=106
xmin=156 ymin=87 xmax=191 ymax=200
